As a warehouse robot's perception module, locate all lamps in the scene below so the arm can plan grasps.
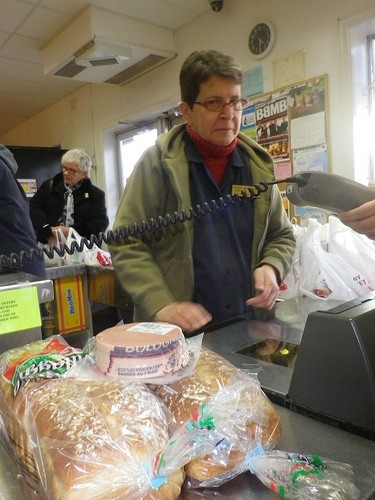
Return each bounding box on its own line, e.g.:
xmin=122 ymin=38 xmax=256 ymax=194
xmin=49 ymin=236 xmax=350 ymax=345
xmin=45 ymin=7 xmax=178 ymax=88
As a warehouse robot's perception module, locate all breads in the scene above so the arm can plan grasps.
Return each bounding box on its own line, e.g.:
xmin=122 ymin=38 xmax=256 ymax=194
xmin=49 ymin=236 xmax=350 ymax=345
xmin=89 ymin=322 xmax=281 ymax=482
xmin=0 ymin=340 xmax=186 ymax=500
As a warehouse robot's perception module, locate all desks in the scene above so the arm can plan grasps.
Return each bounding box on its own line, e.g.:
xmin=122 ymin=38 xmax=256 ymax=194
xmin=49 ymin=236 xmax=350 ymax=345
xmin=0 ymin=310 xmax=375 ymax=500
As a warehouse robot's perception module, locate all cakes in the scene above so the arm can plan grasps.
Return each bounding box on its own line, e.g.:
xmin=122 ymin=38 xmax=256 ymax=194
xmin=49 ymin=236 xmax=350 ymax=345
xmin=95 ymin=322 xmax=191 ymax=379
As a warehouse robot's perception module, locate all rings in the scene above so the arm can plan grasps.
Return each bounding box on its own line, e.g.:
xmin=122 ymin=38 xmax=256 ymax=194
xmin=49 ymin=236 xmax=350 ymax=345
xmin=271 ymin=300 xmax=276 ymax=303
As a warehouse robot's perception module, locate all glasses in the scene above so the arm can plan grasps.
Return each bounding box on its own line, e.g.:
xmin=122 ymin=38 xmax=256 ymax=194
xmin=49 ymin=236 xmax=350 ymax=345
xmin=61 ymin=165 xmax=82 ymax=175
xmin=189 ymin=98 xmax=249 ymax=113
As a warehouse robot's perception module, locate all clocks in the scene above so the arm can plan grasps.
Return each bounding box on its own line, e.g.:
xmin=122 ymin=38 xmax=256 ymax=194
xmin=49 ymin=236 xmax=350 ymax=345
xmin=247 ymin=22 xmax=274 ymax=59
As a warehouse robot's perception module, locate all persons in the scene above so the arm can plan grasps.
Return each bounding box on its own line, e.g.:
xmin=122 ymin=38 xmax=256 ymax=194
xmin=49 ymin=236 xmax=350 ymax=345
xmin=337 ymin=181 xmax=375 ymax=244
xmin=0 ymin=143 xmax=50 ymax=341
xmin=29 ymin=148 xmax=109 ymax=253
xmin=111 ymin=48 xmax=297 ymax=343
